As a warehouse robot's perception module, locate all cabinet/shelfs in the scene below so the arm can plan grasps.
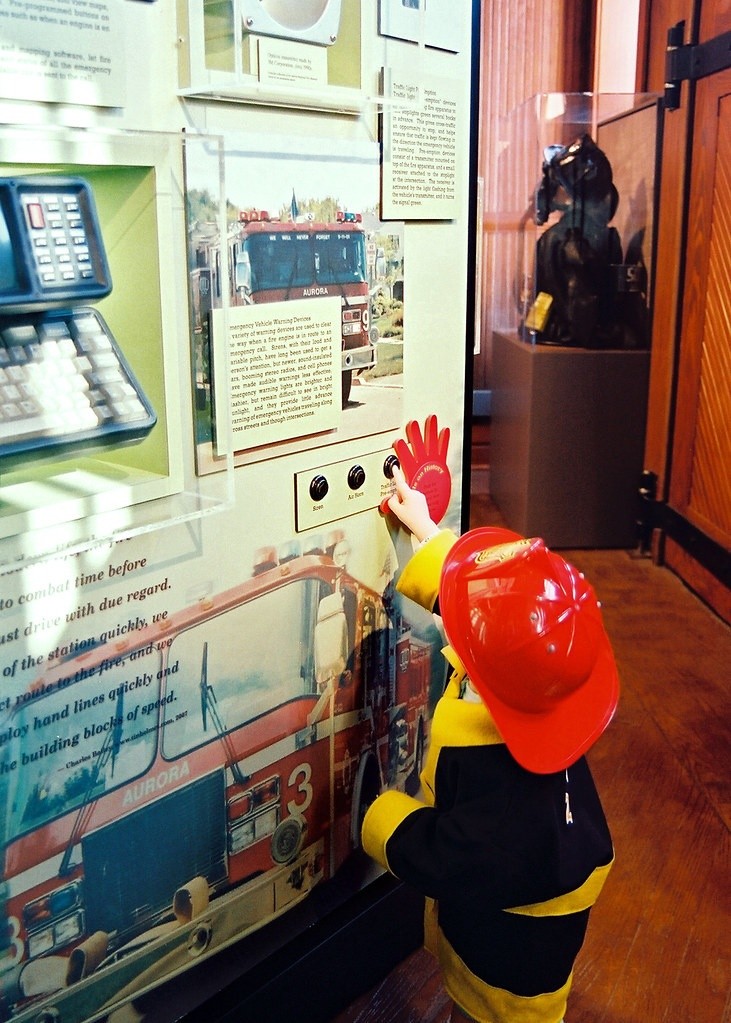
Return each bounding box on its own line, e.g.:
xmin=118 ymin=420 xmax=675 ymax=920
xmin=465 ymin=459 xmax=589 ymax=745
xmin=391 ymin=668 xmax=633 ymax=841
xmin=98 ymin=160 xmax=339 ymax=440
xmin=490 ymin=330 xmax=650 ymax=548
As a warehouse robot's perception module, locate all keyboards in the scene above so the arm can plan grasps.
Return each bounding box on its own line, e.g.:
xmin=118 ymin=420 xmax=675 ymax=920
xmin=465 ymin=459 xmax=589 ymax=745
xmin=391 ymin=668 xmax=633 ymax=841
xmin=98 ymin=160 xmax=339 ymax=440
xmin=0 ymin=307 xmax=157 ymax=467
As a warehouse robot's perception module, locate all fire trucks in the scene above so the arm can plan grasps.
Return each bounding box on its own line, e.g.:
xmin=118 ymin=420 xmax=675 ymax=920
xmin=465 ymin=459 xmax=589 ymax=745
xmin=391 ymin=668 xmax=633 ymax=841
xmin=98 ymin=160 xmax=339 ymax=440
xmin=0 ymin=535 xmax=429 ymax=1023
xmin=183 ymin=187 xmax=378 ymax=405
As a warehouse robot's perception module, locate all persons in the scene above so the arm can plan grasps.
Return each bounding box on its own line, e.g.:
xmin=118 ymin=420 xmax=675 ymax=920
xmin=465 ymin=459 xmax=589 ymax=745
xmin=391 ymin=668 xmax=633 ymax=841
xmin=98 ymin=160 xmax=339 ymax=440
xmin=361 ymin=465 xmax=621 ymax=1023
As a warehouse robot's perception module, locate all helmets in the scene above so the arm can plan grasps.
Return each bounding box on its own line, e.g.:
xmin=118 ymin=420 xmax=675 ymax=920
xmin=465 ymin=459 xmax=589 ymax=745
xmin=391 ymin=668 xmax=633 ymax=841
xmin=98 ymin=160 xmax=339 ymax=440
xmin=438 ymin=526 xmax=620 ymax=774
